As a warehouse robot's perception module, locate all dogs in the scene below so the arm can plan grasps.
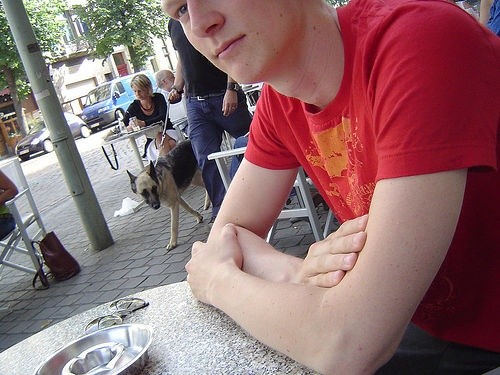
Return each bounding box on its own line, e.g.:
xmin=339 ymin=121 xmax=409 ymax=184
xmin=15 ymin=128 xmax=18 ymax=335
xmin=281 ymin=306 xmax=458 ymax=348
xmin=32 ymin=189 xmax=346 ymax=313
xmin=126 ymin=140 xmax=210 ymax=250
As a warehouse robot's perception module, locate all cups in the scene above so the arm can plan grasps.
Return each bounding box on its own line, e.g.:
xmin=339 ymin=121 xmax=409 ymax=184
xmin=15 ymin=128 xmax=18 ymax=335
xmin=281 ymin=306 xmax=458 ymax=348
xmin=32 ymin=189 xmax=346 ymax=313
xmin=129 ymin=116 xmax=141 ymax=133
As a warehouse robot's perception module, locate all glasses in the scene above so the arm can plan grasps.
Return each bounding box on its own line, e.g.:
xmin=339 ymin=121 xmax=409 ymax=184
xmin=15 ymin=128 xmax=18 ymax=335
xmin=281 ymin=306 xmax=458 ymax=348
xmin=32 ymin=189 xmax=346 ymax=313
xmin=85 ymin=296 xmax=150 ymax=336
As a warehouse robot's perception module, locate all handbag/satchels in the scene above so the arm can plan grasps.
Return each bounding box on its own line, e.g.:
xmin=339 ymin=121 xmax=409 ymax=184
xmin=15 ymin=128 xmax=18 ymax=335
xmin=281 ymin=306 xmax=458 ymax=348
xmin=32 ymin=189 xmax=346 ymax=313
xmin=31 ymin=232 xmax=80 ymax=282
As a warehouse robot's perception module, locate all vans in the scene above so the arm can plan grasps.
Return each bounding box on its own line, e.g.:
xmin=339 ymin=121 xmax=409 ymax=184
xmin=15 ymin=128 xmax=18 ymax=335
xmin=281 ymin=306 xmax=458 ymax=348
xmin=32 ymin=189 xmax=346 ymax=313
xmin=79 ymin=70 xmax=158 ymax=133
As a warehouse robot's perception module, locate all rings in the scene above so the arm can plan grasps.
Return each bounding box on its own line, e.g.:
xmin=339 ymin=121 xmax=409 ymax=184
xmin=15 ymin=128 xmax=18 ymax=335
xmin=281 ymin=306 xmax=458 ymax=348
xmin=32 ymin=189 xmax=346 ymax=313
xmin=232 ymin=107 xmax=235 ymax=109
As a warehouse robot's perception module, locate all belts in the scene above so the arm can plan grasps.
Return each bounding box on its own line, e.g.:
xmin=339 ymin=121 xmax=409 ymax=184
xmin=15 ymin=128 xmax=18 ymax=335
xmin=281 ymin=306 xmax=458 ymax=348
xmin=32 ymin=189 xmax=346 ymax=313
xmin=190 ymin=91 xmax=224 ymax=101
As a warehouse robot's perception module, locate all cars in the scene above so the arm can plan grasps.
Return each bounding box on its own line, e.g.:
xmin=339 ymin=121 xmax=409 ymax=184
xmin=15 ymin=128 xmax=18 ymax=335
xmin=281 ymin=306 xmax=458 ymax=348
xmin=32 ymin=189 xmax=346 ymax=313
xmin=15 ymin=111 xmax=91 ymax=161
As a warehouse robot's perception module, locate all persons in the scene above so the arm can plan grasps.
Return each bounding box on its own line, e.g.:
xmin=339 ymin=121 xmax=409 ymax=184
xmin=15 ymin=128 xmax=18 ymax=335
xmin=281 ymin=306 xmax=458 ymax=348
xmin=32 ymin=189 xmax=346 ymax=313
xmin=155 ymin=69 xmax=175 ymax=97
xmin=160 ymin=0 xmax=500 ymax=375
xmin=166 ymin=18 xmax=303 ymax=228
xmin=487 ymin=0 xmax=500 ymax=35
xmin=0 ymin=171 xmax=18 ymax=240
xmin=123 ymin=74 xmax=179 ymax=157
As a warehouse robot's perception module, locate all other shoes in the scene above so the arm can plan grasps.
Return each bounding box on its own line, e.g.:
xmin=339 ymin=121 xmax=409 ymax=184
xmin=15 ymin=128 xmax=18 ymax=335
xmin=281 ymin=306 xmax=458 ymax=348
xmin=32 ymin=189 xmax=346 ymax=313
xmin=207 ymin=217 xmax=214 ymax=229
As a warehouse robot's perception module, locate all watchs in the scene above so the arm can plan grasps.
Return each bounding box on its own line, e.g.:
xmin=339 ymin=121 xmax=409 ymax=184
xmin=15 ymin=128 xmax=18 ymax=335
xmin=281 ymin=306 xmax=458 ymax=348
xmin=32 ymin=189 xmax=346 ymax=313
xmin=228 ymin=82 xmax=239 ymax=91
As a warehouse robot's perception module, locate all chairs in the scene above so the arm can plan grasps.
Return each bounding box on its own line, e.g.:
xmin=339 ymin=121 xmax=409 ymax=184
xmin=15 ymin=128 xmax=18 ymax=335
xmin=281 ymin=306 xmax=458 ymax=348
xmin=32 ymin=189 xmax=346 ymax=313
xmin=0 ymin=157 xmax=50 ymax=289
xmin=208 ymin=143 xmax=334 ymax=244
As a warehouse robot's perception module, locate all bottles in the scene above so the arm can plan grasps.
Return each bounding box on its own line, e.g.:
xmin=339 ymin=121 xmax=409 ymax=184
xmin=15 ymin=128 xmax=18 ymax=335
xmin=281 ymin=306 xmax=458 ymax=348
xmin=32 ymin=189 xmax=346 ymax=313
xmin=118 ymin=118 xmax=127 ymax=134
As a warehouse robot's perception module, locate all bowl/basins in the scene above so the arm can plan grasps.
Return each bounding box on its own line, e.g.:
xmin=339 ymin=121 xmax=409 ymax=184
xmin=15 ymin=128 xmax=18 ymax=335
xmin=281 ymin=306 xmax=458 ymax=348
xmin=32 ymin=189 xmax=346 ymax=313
xmin=33 ymin=323 xmax=154 ymax=375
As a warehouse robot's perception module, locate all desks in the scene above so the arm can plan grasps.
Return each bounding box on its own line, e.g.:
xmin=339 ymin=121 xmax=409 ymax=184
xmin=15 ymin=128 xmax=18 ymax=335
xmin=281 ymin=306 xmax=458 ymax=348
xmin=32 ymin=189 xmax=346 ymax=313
xmin=105 ymin=123 xmax=160 ymax=172
xmin=0 ymin=280 xmax=321 ymax=375
